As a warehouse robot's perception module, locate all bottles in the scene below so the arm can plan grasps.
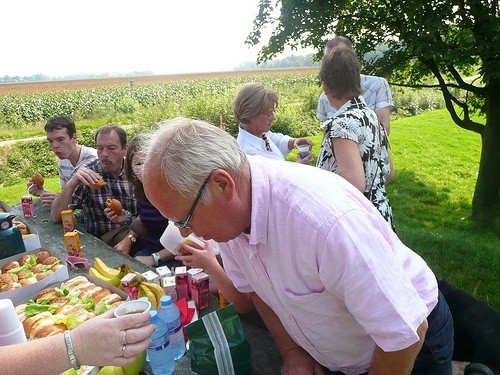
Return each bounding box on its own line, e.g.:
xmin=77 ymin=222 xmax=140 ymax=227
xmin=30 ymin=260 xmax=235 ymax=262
xmin=148 ymin=311 xmax=175 ymax=375
xmin=158 ymin=296 xmax=186 ymax=360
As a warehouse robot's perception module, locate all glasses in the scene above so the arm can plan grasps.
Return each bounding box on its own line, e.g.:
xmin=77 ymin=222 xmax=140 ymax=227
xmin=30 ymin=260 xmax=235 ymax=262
xmin=173 ymin=168 xmax=215 ymax=230
xmin=259 ymin=112 xmax=274 ymax=120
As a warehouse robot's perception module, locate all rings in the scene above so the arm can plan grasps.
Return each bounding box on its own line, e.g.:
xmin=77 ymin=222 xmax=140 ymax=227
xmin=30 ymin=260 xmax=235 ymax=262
xmin=122 ymin=331 xmax=128 ymax=345
xmin=119 ymin=347 xmax=126 ymax=357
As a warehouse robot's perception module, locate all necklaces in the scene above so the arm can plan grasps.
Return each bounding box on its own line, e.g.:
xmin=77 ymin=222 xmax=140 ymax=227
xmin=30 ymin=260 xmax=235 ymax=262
xmin=244 ymin=126 xmax=273 ymax=153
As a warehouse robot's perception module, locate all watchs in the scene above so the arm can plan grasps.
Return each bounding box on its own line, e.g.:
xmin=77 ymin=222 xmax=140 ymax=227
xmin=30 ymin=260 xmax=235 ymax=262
xmin=152 ymin=252 xmax=161 ymax=266
xmin=128 ymin=234 xmax=137 ymax=243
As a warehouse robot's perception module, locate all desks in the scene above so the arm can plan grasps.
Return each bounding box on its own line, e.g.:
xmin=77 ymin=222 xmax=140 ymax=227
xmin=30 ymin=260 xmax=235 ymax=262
xmin=9 ymin=197 xmax=283 ymax=375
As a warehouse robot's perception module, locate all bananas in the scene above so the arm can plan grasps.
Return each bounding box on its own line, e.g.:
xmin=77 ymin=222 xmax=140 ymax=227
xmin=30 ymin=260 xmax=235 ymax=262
xmin=89 ymin=257 xmax=165 ymax=309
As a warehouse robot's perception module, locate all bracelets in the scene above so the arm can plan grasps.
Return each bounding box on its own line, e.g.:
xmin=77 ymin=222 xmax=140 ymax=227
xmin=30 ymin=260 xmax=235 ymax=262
xmin=122 ymin=210 xmax=132 ymax=225
xmin=64 ymin=329 xmax=80 ymax=370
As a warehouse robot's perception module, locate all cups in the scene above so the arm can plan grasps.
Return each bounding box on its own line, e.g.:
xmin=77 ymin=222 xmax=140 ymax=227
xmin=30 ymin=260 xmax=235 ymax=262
xmin=114 ymin=300 xmax=152 ymax=329
xmin=298 ymin=145 xmax=309 ymax=158
xmin=0 ymin=299 xmax=27 ymax=345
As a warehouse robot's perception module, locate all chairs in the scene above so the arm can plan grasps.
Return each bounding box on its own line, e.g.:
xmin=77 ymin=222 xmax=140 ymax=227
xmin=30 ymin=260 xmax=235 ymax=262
xmin=437 ymin=279 xmax=500 ymax=375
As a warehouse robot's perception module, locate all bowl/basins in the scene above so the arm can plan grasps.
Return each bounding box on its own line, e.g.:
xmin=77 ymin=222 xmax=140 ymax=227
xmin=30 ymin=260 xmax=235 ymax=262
xmin=159 ymin=220 xmax=220 ymax=259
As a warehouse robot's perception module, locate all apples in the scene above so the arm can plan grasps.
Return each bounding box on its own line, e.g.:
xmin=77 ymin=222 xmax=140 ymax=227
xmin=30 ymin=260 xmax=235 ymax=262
xmin=98 ymin=347 xmax=146 ymax=375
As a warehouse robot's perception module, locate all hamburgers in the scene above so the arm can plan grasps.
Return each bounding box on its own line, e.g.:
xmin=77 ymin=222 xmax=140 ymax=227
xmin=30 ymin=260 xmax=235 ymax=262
xmin=0 ymin=251 xmax=123 ymax=341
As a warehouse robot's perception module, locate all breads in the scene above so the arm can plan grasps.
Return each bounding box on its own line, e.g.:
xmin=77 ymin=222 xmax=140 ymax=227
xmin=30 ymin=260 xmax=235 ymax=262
xmin=106 ymin=198 xmax=122 ymax=215
xmin=89 ymin=173 xmax=107 ymax=189
xmin=30 ymin=173 xmax=43 ymax=187
xmin=12 ymin=221 xmax=37 ymax=240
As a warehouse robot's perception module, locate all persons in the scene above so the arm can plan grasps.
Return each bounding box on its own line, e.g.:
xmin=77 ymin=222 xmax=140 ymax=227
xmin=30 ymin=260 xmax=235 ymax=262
xmin=51 ymin=126 xmax=139 ymax=238
xmin=317 ymin=36 xmax=395 ymax=136
xmin=101 ymin=133 xmax=256 ymax=316
xmin=316 ymin=47 xmax=395 ymax=232
xmin=234 ymin=84 xmax=314 ymax=164
xmin=143 ymin=117 xmax=454 ymax=375
xmin=27 ymin=115 xmax=98 ymax=209
xmin=1 ymin=306 xmax=156 ymax=375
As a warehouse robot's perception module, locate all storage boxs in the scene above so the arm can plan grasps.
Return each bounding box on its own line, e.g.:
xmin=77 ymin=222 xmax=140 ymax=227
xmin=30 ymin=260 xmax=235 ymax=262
xmin=0 ymin=247 xmax=69 ymax=306
xmin=14 ymin=272 xmax=130 ymax=309
xmin=0 ymin=201 xmax=41 ymax=251
